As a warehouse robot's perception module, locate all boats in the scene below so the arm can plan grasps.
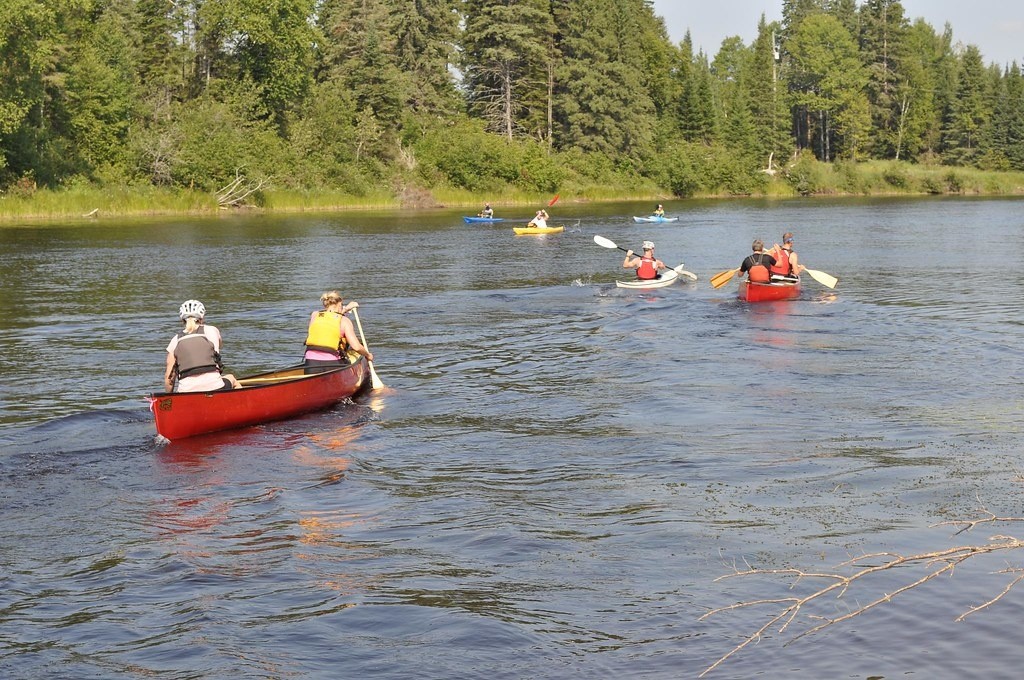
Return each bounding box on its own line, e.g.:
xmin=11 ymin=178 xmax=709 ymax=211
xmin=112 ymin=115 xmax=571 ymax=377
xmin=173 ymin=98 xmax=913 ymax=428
xmin=739 ymin=274 xmax=802 ymax=302
xmin=151 ymin=343 xmax=369 ymax=440
xmin=615 ymin=263 xmax=685 ymax=289
xmin=463 ymin=216 xmax=504 ymax=224
xmin=513 ymin=226 xmax=564 ymax=235
xmin=632 ymin=215 xmax=679 ymax=222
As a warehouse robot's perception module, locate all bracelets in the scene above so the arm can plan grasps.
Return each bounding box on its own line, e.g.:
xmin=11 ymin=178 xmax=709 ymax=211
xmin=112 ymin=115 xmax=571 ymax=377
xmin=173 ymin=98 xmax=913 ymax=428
xmin=626 ymin=255 xmax=630 ymax=258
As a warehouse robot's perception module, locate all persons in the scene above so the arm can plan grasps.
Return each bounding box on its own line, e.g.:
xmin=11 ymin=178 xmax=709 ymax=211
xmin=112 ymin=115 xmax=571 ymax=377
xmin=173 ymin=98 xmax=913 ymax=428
xmin=769 ymin=232 xmax=805 ymax=282
xmin=481 ymin=204 xmax=493 ymax=219
xmin=164 ymin=299 xmax=243 ymax=392
xmin=738 ymin=240 xmax=783 ymax=286
xmin=531 ymin=210 xmax=549 ymax=229
xmin=653 ymin=204 xmax=665 ymax=218
xmin=303 ymin=291 xmax=373 ymax=375
xmin=623 ymin=241 xmax=664 ymax=281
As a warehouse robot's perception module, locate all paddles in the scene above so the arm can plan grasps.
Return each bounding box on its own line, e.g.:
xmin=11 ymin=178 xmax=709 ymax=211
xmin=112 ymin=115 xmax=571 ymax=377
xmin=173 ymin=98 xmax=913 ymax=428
xmin=763 ymin=248 xmax=837 ymax=289
xmin=710 ymin=248 xmax=775 ymax=288
xmin=531 ymin=195 xmax=560 ymax=223
xmin=353 ymin=307 xmax=384 ymax=388
xmin=594 ymin=235 xmax=697 ymax=280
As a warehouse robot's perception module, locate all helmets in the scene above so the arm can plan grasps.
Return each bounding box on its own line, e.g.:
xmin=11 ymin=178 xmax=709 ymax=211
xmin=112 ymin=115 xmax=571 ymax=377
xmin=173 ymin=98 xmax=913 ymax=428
xmin=179 ymin=300 xmax=206 ymax=319
xmin=642 ymin=241 xmax=655 ymax=251
